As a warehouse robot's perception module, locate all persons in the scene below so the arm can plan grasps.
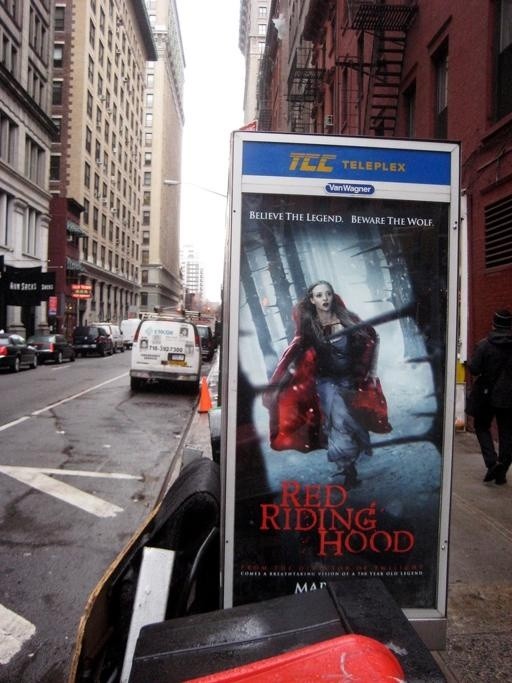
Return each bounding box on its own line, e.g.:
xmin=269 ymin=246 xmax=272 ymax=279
xmin=288 ymin=281 xmax=377 ymax=488
xmin=463 ymin=309 xmax=512 ymax=485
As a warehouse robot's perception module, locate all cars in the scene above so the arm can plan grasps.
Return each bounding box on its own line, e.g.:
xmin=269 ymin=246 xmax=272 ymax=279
xmin=0 ymin=332 xmax=39 ymax=373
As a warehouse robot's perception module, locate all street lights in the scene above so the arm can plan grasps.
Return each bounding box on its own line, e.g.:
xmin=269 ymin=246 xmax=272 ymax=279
xmin=164 ymin=178 xmax=229 ymax=202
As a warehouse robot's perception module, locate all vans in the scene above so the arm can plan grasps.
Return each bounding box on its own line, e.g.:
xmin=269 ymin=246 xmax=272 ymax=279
xmin=129 ymin=318 xmax=201 ymax=397
xmin=196 ymin=324 xmax=213 ymax=360
xmin=119 ymin=318 xmax=141 ymax=345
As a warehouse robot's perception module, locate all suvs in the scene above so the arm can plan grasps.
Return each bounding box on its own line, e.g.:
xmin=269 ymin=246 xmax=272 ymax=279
xmin=27 ymin=334 xmax=76 ymax=364
xmin=92 ymin=322 xmax=123 ymax=353
xmin=71 ymin=326 xmax=114 ymax=357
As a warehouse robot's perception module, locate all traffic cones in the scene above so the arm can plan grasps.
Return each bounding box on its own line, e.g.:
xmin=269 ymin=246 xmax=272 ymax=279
xmin=198 ymin=376 xmax=215 ymax=414
